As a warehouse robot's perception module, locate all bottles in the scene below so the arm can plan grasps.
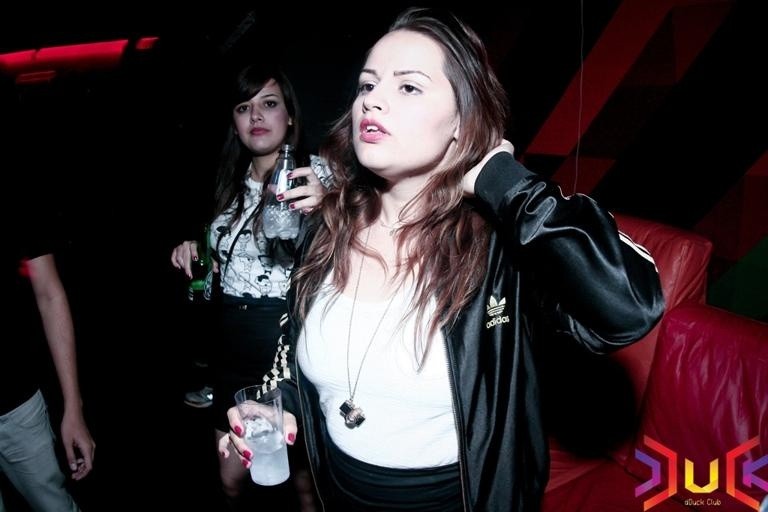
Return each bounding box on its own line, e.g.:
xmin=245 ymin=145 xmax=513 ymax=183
xmin=187 ymin=226 xmax=212 ymax=313
xmin=262 ymin=145 xmax=301 ymax=240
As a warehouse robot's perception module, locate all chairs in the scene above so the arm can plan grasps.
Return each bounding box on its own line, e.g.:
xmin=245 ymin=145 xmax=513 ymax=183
xmin=542 ymin=304 xmax=768 ymax=512
xmin=545 ymin=215 xmax=712 ymax=493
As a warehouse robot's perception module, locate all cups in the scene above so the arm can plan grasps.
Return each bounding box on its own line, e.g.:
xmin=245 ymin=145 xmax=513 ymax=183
xmin=234 ymin=385 xmax=292 ymax=487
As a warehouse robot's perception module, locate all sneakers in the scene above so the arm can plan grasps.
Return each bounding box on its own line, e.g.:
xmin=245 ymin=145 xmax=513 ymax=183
xmin=184 ymin=386 xmax=213 ymax=409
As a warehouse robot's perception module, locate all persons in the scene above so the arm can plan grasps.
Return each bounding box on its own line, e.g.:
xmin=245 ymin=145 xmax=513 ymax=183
xmin=0 ymin=179 xmax=96 ymax=512
xmin=215 ymin=5 xmax=666 ymax=509
xmin=170 ymin=62 xmax=357 ymax=511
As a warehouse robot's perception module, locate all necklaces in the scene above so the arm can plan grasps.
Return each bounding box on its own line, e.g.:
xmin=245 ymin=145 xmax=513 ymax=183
xmin=336 ymin=219 xmax=424 ymax=427
